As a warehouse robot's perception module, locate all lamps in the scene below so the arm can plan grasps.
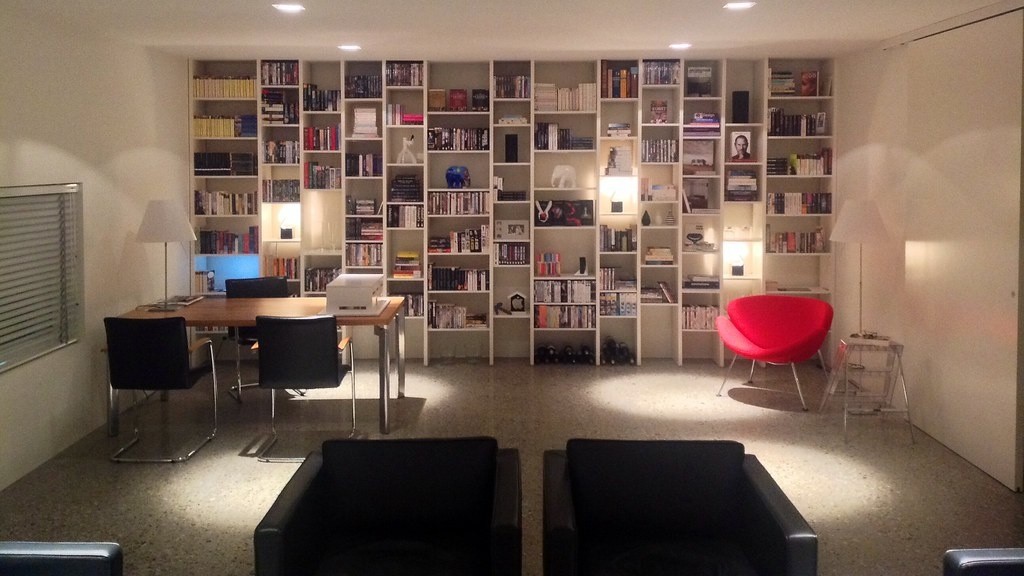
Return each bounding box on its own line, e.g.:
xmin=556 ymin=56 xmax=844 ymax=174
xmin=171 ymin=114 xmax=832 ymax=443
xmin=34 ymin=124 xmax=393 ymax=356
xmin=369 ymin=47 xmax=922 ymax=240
xmin=829 ymin=197 xmax=890 ymax=417
xmin=137 ymin=200 xmax=197 ymax=312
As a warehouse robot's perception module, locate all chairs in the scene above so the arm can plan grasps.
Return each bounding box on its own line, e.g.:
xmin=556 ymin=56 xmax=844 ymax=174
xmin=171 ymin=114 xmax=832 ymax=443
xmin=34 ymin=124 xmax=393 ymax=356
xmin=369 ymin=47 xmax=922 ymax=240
xmin=103 ymin=317 xmax=218 ymax=463
xmin=254 ymin=315 xmax=356 ymax=463
xmin=225 ymin=276 xmax=306 ymax=403
xmin=252 ymin=436 xmax=524 ymax=576
xmin=542 ymin=438 xmax=819 ymax=576
xmin=715 ymin=294 xmax=834 ymax=412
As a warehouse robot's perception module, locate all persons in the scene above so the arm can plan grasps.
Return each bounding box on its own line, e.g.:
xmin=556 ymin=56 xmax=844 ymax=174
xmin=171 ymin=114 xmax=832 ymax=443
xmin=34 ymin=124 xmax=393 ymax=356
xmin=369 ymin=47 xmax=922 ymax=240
xmin=731 ymin=135 xmax=750 ymax=160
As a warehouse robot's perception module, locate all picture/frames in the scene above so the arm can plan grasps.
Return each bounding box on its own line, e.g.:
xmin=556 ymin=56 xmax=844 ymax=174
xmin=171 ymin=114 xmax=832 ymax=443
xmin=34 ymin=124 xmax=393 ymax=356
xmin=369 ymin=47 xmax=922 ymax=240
xmin=730 ymin=130 xmax=753 ymax=162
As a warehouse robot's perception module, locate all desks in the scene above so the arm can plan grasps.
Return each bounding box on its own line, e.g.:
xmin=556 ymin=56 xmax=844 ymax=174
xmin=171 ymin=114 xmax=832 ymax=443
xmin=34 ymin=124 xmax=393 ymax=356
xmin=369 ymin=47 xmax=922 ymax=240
xmin=116 ymin=295 xmax=406 ymax=434
xmin=818 ymin=337 xmax=915 ymax=446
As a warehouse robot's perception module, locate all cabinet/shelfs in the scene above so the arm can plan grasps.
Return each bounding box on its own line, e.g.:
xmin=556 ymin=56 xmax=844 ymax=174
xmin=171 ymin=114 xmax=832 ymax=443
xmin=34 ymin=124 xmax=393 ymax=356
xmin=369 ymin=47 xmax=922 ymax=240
xmin=189 ymin=59 xmax=838 ymax=368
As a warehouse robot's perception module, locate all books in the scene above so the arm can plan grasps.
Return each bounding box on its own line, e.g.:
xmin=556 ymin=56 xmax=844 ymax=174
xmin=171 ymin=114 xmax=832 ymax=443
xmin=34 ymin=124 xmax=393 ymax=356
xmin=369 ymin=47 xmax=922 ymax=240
xmin=189 ymin=59 xmax=835 ymax=374
xmin=166 ymin=296 xmax=203 ymax=304
